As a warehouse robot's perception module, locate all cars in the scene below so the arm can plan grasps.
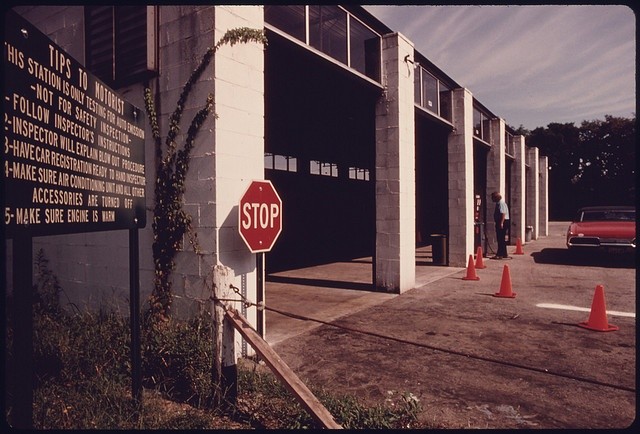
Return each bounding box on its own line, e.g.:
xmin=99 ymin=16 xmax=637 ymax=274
xmin=566 ymin=206 xmax=637 ymax=255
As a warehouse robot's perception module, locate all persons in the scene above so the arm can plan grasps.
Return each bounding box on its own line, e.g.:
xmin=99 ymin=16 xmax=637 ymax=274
xmin=491 ymin=191 xmax=510 ymax=259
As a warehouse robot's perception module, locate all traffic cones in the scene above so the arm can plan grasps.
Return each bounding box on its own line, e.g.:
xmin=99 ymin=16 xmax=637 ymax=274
xmin=475 ymin=247 xmax=485 ymax=268
xmin=462 ymin=255 xmax=480 ymax=280
xmin=514 ymin=238 xmax=524 ymax=254
xmin=578 ymin=285 xmax=618 ymax=331
xmin=494 ymin=265 xmax=517 ymax=298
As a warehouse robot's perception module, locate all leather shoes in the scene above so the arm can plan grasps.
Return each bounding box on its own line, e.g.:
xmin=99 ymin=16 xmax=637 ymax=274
xmin=490 ymin=256 xmax=502 ymax=260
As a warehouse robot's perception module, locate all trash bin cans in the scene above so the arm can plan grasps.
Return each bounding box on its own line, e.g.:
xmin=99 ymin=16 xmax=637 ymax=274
xmin=430 ymin=233 xmax=449 ymax=266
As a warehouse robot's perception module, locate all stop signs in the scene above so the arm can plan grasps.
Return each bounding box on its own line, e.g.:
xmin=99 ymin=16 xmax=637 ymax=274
xmin=238 ymin=180 xmax=282 ymax=253
